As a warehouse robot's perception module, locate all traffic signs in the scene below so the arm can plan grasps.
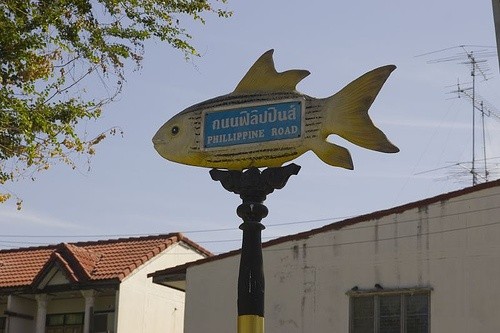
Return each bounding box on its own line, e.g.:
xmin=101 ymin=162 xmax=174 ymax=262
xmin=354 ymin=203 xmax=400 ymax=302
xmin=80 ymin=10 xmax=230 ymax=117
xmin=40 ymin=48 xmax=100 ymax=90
xmin=199 ymin=95 xmax=307 ymax=155
